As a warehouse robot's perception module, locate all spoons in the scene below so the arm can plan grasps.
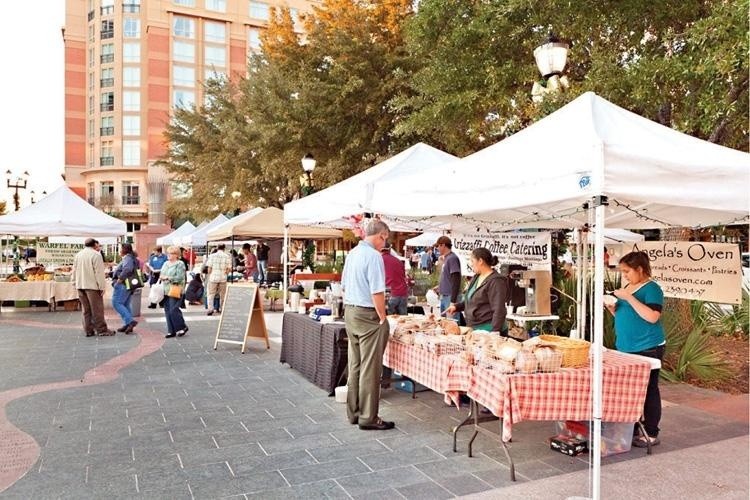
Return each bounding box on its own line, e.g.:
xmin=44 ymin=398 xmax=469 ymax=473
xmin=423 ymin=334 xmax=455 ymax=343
xmin=605 ymin=291 xmax=614 ymax=294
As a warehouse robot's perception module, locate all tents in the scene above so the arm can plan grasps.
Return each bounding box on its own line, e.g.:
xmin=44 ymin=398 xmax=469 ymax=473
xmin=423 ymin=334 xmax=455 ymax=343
xmin=451 ymin=89 xmax=750 ymax=499
xmin=179 ymin=214 xmax=231 ymax=270
xmin=157 ymin=220 xmax=196 ymax=248
xmin=175 ymin=221 xmax=213 ymax=250
xmin=0 ymin=184 xmax=127 ymax=313
xmin=206 ymin=207 xmax=344 ymax=336
xmin=283 ymin=143 xmax=461 ymax=353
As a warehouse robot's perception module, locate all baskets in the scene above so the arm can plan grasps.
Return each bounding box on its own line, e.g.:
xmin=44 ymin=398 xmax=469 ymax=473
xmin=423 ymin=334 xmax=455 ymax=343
xmin=538 ymin=335 xmax=590 ymax=368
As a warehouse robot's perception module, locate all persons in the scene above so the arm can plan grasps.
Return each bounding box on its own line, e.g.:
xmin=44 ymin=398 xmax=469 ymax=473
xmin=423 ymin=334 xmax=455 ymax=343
xmin=445 ymin=248 xmax=508 ymax=413
xmin=146 ymin=247 xmax=168 ymax=308
xmin=186 ymin=274 xmax=204 ymax=305
xmin=81 ymin=241 xmax=105 ymax=330
xmin=433 ymin=236 xmax=462 ymax=326
xmin=201 ymin=248 xmax=220 ymax=311
xmin=605 ymin=252 xmax=666 ymax=447
xmin=380 ymin=243 xmax=407 ymax=315
xmin=237 ymin=243 xmax=259 ymax=282
xmin=409 ymin=247 xmax=439 ymax=270
xmin=159 ymin=246 xmax=188 ymax=338
xmin=72 ymin=239 xmax=116 ymax=336
xmin=342 ymin=219 xmax=394 ymax=429
xmin=231 ymin=249 xmax=248 ymax=281
xmin=256 ymin=238 xmax=272 ymax=286
xmin=179 ymin=247 xmax=188 ymax=309
xmin=105 ymin=244 xmax=138 ymax=334
xmin=206 ymin=243 xmax=232 ymax=315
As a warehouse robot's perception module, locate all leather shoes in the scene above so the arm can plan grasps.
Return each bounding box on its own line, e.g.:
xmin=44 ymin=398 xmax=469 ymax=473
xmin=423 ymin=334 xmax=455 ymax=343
xmin=166 ymin=332 xmax=176 ymax=337
xmin=178 ymin=327 xmax=188 ymax=336
xmin=360 ymin=418 xmax=396 ymax=429
xmin=634 ymin=437 xmax=660 ymax=447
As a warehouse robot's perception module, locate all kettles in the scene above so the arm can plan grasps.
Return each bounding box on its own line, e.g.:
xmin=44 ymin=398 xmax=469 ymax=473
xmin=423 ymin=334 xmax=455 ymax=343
xmin=287 ymin=284 xmax=304 ymax=314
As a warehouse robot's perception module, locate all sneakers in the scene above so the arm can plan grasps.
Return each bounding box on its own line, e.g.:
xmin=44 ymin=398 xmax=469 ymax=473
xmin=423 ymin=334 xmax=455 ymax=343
xmin=86 ymin=332 xmax=95 ymax=337
xmin=125 ymin=321 xmax=137 ymax=333
xmin=97 ymin=330 xmax=116 ymax=336
xmin=119 ymin=325 xmax=133 ymax=332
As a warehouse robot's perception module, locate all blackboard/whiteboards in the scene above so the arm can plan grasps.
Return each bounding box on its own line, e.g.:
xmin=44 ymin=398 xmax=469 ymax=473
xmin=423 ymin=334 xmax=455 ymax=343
xmin=218 ymin=286 xmax=255 ymax=343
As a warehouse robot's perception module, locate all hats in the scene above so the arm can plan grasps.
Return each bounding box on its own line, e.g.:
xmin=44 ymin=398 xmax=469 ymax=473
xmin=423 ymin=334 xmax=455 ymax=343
xmin=432 ymin=236 xmax=452 ymax=246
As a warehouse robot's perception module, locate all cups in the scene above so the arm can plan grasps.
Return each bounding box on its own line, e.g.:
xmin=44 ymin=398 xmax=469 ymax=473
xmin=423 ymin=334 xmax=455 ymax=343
xmin=516 ymin=305 xmax=527 ymax=315
xmin=446 ymin=313 xmax=454 ymax=321
xmin=423 ymin=306 xmax=432 ymax=316
xmin=433 ymin=307 xmax=441 ymax=319
xmin=505 ymin=302 xmax=514 ymax=316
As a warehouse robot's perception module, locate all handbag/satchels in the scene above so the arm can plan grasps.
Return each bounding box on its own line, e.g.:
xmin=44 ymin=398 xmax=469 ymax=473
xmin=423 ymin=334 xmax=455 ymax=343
xmin=123 ymin=255 xmax=144 ymax=290
xmin=168 ymin=282 xmax=181 ymax=298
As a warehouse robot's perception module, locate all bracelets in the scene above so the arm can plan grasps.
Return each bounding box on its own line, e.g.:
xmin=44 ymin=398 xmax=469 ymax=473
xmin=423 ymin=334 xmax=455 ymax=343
xmin=167 ymin=276 xmax=169 ymax=280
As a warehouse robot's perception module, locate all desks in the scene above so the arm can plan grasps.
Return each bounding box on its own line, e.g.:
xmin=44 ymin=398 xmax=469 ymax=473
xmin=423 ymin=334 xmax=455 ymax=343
xmin=380 ymin=322 xmax=508 ymax=452
xmin=52 ymin=282 xmax=79 ymax=312
xmin=468 ymin=346 xmax=661 ymax=482
xmin=0 ymin=282 xmax=51 ymax=312
xmin=280 ymin=311 xmax=349 ymax=397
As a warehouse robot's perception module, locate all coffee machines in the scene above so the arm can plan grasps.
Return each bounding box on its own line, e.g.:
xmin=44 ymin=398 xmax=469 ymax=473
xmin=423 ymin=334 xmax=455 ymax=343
xmin=510 ymin=270 xmax=551 ymax=317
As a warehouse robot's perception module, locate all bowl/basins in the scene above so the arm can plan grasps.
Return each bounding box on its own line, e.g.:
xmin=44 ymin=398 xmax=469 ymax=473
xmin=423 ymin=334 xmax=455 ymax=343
xmin=603 ymin=296 xmax=617 ymax=305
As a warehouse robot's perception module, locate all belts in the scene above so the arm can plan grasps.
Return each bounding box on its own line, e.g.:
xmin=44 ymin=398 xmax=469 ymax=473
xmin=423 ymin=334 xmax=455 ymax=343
xmin=350 ymin=305 xmax=375 ymax=310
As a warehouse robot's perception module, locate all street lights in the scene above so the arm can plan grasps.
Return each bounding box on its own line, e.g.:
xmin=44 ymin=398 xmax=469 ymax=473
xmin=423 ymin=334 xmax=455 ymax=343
xmin=27 ymin=187 xmax=47 ymax=206
xmin=6 ymin=170 xmax=32 ymax=189
xmin=231 ymin=187 xmax=242 ymax=217
xmin=300 ymin=152 xmax=316 ymax=196
xmin=532 ymin=35 xmax=570 ymax=81
